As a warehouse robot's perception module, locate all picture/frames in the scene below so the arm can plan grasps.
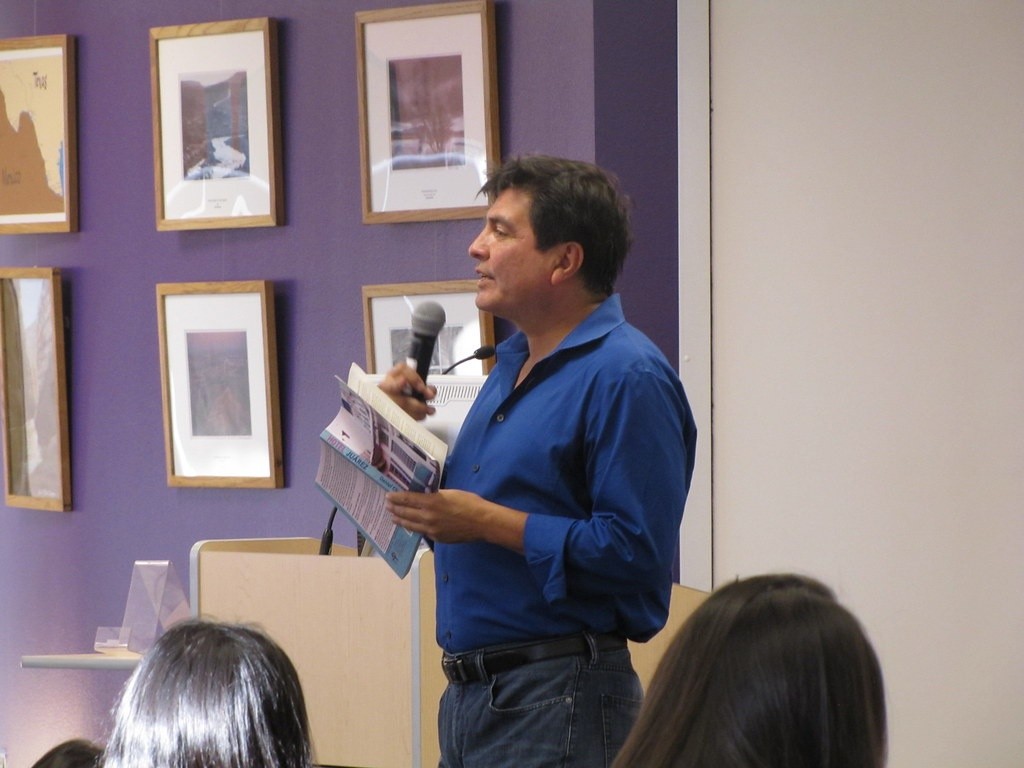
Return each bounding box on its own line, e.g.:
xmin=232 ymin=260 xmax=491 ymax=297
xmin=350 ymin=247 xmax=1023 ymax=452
xmin=0 ymin=266 xmax=73 ymax=513
xmin=0 ymin=34 xmax=78 ymax=235
xmin=355 ymin=0 xmax=501 ymax=226
xmin=362 ymin=279 xmax=497 ymax=376
xmin=148 ymin=17 xmax=287 ymax=234
xmin=156 ymin=280 xmax=283 ymax=490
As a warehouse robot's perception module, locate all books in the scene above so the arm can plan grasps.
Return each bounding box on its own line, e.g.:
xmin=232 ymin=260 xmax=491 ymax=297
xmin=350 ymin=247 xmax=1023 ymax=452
xmin=314 ymin=364 xmax=449 ymax=578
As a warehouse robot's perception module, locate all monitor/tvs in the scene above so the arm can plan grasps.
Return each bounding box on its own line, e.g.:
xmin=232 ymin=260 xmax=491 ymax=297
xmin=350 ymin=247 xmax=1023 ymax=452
xmin=367 ymin=373 xmax=490 ymax=459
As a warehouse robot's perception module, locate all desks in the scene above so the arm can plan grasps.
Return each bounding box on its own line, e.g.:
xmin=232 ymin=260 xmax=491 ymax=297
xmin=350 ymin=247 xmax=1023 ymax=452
xmin=19 ymin=647 xmax=143 ymax=671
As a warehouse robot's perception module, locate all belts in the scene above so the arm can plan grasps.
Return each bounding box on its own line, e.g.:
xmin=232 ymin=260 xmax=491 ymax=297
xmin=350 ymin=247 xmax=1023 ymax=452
xmin=439 ymin=634 xmax=628 ymax=685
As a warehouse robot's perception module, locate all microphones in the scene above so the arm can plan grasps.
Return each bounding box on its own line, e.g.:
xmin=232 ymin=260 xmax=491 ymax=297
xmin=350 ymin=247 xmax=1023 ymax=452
xmin=442 ymin=345 xmax=495 ymax=374
xmin=401 ymin=301 xmax=446 ymax=403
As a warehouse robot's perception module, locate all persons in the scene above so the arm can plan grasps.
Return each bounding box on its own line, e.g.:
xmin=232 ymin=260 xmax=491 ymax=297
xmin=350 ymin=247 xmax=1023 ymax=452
xmin=378 ymin=153 xmax=698 ymax=768
xmin=610 ymin=573 xmax=891 ymax=768
xmin=99 ymin=615 xmax=317 ymax=768
